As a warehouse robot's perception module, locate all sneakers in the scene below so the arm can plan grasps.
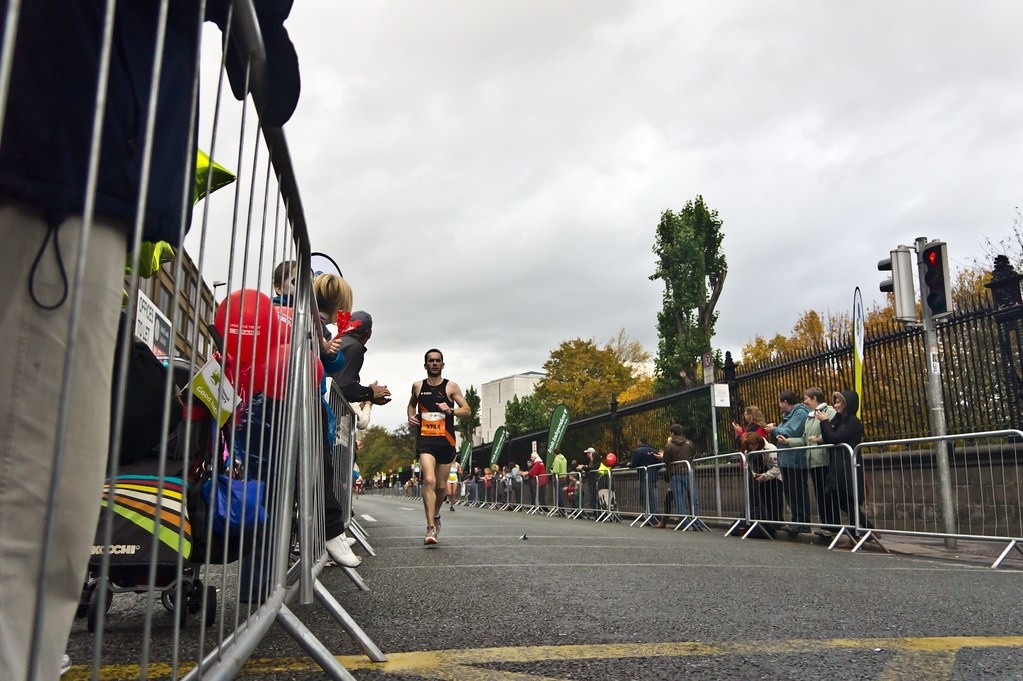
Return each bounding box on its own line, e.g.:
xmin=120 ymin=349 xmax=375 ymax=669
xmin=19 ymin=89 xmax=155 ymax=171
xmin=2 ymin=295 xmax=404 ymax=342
xmin=814 ymin=529 xmax=838 ymax=537
xmin=434 ymin=514 xmax=441 ymax=537
xmin=424 ymin=526 xmax=438 ymax=545
xmin=326 ymin=532 xmax=362 ymax=568
xmin=450 ymin=507 xmax=455 ymax=511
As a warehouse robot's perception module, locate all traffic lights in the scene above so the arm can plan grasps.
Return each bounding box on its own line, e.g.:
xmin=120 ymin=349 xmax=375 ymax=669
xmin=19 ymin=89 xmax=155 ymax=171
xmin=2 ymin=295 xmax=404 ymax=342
xmin=877 ymin=244 xmax=918 ymax=330
xmin=920 ymin=237 xmax=955 ymax=323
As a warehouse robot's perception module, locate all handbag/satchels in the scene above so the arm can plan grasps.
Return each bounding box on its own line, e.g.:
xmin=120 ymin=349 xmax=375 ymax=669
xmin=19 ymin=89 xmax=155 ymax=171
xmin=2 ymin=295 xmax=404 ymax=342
xmin=186 ymin=467 xmax=253 ymax=562
xmin=202 ymin=473 xmax=270 ymax=538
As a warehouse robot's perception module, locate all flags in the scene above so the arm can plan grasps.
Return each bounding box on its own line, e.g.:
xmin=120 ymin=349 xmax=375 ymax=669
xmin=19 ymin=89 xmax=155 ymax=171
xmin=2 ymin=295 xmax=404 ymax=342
xmin=460 ymin=440 xmax=471 ymax=470
xmin=490 ymin=426 xmax=506 ymax=465
xmin=854 ymin=287 xmax=863 ymax=421
xmin=546 ymin=404 xmax=570 ymax=471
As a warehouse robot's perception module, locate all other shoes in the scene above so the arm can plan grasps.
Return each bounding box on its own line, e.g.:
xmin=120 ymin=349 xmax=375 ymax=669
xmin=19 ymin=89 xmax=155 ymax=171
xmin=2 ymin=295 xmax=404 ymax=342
xmin=837 ymin=539 xmax=862 ymax=549
xmin=865 ymin=531 xmax=881 ymax=539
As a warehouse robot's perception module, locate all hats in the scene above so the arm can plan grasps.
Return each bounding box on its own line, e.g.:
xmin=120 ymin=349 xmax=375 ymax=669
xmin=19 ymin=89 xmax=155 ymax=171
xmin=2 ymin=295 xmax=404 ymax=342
xmin=349 ymin=311 xmax=372 ymax=329
xmin=584 ymin=447 xmax=596 ymax=453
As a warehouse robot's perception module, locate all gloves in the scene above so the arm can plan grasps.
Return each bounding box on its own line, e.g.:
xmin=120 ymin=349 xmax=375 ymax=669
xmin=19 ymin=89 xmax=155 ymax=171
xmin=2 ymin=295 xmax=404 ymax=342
xmin=223 ymin=23 xmax=300 ymax=128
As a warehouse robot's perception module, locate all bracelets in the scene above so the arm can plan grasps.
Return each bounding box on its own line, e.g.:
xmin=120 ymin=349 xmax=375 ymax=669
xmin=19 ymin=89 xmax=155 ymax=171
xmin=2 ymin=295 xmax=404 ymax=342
xmin=450 ymin=408 xmax=454 ymax=415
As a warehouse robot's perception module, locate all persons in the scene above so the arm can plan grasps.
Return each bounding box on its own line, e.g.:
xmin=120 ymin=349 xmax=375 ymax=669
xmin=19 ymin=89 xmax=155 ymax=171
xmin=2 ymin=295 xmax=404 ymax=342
xmin=627 ymin=424 xmax=700 ymax=531
xmin=407 ymin=349 xmax=472 ymax=545
xmin=446 ymin=457 xmax=462 ymax=511
xmin=408 ymin=476 xmax=418 ymax=499
xmin=731 ymin=388 xmax=881 ymax=541
xmin=0 ymin=0 xmax=391 ymax=681
xmin=474 ymin=448 xmax=613 ymax=521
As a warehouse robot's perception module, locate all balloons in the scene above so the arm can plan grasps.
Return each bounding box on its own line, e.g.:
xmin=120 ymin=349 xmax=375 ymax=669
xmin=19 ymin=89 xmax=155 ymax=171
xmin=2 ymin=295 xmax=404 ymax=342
xmin=274 ymin=307 xmax=293 ymax=344
xmin=215 ymin=288 xmax=277 ymax=364
xmin=255 ymin=345 xmax=324 ymax=400
xmin=605 ymin=453 xmax=616 ymax=465
